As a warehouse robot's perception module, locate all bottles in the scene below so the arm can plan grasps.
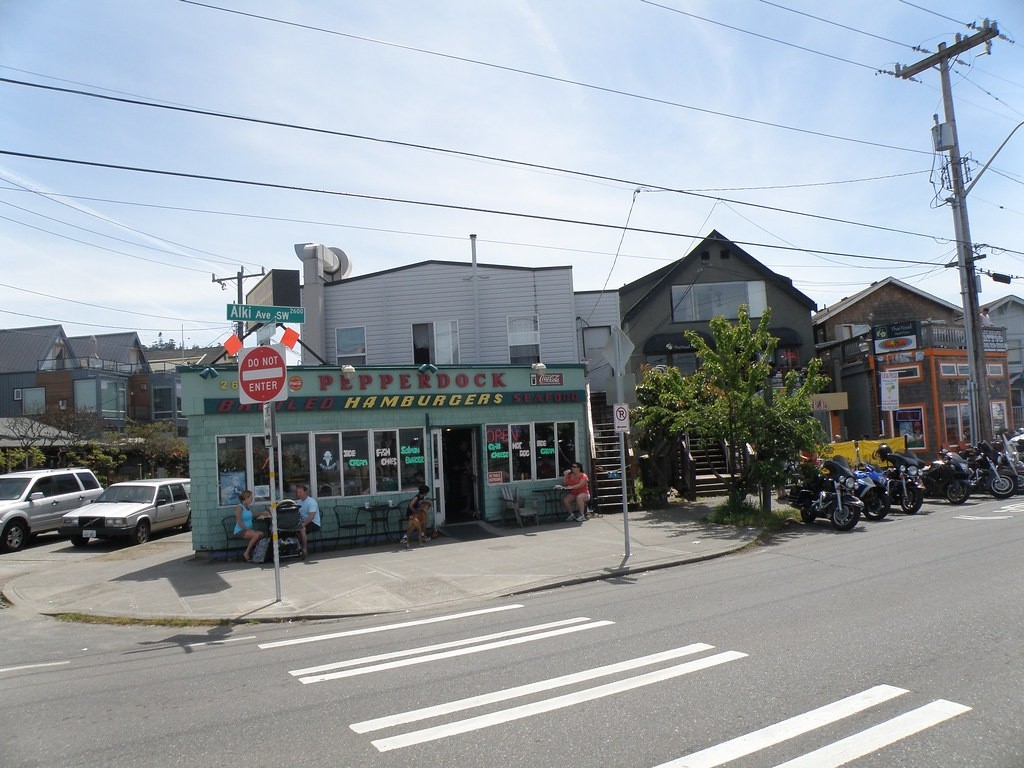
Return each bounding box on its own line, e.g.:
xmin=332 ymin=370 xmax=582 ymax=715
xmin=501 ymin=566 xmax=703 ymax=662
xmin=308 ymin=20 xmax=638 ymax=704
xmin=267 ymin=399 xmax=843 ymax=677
xmin=564 ymin=470 xmax=571 ymax=474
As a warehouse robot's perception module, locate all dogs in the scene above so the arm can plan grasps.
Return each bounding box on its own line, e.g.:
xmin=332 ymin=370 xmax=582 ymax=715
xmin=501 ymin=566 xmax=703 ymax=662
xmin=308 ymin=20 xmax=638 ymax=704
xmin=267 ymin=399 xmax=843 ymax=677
xmin=404 ymin=499 xmax=430 ymax=549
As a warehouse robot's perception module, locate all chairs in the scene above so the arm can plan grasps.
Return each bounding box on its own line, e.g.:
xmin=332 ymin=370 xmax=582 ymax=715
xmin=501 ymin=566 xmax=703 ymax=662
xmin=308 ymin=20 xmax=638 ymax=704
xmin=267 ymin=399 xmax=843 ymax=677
xmin=396 ymin=499 xmax=411 ymax=540
xmin=156 ymin=490 xmax=170 ymax=506
xmin=568 ymin=481 xmax=597 ymax=517
xmin=304 ymin=509 xmax=325 ymax=552
xmin=334 ymin=505 xmax=368 ymax=548
xmin=501 ymin=486 xmax=540 ymax=528
xmin=221 ymin=515 xmax=253 ymax=559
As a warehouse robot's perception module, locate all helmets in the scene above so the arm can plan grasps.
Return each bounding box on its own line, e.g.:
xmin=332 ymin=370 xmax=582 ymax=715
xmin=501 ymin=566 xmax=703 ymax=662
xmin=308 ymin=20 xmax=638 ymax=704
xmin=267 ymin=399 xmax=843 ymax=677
xmin=876 ymin=445 xmax=892 ymax=461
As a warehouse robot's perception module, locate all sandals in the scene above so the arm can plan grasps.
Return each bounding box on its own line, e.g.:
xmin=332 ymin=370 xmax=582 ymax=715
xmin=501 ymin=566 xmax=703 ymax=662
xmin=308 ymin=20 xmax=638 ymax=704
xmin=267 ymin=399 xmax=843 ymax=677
xmin=243 ymin=552 xmax=253 ymax=564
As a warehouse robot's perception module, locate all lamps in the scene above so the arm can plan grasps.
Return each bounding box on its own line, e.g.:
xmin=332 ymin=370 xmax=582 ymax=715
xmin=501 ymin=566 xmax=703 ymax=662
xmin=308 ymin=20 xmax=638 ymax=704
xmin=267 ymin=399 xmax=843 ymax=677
xmin=418 ymin=364 xmax=428 ymax=373
xmin=341 ymin=365 xmax=357 ymax=380
xmin=858 ymin=336 xmax=871 ymax=357
xmin=428 ymin=364 xmax=439 ymax=373
xmin=200 ymin=367 xmax=209 ymax=381
xmin=532 ymin=363 xmax=547 ymax=376
xmin=208 ymin=365 xmax=219 ymax=379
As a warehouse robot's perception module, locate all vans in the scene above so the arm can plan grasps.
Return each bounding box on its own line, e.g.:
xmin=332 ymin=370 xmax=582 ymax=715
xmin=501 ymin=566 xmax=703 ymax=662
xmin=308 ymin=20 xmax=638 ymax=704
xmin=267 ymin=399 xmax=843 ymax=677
xmin=0 ymin=467 xmax=105 ymax=554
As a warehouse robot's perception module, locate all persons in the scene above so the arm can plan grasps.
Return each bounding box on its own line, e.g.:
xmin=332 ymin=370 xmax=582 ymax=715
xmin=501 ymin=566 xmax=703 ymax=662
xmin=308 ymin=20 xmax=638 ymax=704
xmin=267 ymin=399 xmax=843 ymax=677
xmin=295 ymin=485 xmax=321 ymax=560
xmin=400 ymin=485 xmax=431 ymax=545
xmin=233 ymin=491 xmax=271 ymax=562
xmin=979 ymin=308 xmax=993 ymax=327
xmin=798 ymin=446 xmax=821 ymax=468
xmin=906 ymin=421 xmax=923 ymax=444
xmin=561 ymin=463 xmax=591 ymax=522
xmin=851 ymin=433 xmax=870 ymax=471
xmin=831 ymin=434 xmax=842 ymax=444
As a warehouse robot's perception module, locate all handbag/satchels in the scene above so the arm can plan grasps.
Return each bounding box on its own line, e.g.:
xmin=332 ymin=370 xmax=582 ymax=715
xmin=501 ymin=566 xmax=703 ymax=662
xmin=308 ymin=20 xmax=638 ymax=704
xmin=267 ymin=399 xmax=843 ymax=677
xmin=252 ymin=536 xmax=271 ymax=564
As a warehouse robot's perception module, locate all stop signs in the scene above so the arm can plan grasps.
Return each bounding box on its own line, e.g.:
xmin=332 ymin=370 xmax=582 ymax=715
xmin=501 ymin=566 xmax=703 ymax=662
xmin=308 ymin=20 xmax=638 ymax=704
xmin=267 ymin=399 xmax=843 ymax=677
xmin=238 ymin=346 xmax=287 ymax=403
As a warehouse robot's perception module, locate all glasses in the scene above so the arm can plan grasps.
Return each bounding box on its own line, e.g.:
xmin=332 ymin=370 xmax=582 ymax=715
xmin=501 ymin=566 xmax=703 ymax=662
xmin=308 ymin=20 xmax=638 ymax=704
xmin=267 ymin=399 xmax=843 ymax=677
xmin=571 ymin=465 xmax=578 ymax=468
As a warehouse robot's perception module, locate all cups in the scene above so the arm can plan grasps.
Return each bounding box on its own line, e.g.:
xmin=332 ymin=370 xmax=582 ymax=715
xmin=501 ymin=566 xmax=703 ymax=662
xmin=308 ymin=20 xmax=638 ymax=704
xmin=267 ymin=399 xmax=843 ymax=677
xmin=364 ymin=502 xmax=369 ymax=508
xmin=388 ymin=500 xmax=392 ymax=506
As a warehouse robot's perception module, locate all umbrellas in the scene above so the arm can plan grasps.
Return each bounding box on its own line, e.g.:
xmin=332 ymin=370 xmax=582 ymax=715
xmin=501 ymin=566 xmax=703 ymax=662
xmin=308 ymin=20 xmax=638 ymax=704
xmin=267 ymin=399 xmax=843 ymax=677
xmin=89 ymin=334 xmax=99 ymax=367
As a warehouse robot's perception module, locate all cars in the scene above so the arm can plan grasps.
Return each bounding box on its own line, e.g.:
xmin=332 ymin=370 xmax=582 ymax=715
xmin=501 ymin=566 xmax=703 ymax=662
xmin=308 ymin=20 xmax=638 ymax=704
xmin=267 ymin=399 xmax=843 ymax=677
xmin=57 ymin=478 xmax=192 ymax=548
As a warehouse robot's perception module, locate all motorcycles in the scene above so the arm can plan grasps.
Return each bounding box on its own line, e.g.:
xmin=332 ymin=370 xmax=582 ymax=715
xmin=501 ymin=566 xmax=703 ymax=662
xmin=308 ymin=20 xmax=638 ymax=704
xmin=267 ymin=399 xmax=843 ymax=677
xmin=912 ymin=448 xmax=974 ymax=505
xmin=938 ymin=432 xmax=1024 ymax=500
xmin=820 ymin=452 xmax=891 ymax=522
xmin=799 ymin=453 xmax=866 ymax=531
xmin=869 ymin=448 xmax=927 ymax=515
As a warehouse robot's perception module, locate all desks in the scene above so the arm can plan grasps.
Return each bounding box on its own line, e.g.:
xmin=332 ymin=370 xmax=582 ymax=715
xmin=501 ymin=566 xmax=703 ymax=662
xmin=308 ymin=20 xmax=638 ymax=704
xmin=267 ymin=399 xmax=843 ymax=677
xmin=531 ymin=487 xmax=572 ymax=523
xmin=357 ymin=504 xmax=399 ymax=545
xmin=256 ymin=512 xmax=272 ymax=539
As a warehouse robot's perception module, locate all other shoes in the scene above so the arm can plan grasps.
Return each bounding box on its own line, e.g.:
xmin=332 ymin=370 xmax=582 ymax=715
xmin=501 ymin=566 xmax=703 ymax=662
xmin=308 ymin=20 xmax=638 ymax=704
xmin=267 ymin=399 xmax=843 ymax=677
xmin=778 ymin=494 xmax=788 ymax=499
xmin=577 ymin=514 xmax=585 ymax=521
xmin=567 ymin=514 xmax=576 ymax=522
xmin=422 ymin=535 xmax=430 ymax=541
xmin=400 ymin=537 xmax=409 ymax=543
